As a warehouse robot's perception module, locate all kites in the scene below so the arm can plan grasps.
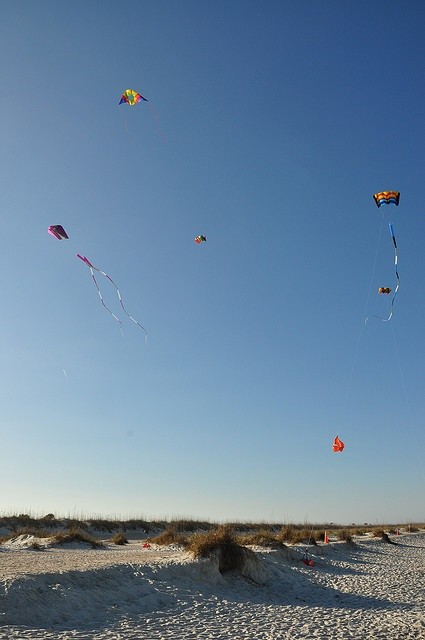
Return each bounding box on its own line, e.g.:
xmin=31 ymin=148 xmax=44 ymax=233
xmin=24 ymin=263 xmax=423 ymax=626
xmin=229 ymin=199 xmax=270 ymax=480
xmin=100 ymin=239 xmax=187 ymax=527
xmin=116 ymin=86 xmax=148 ymax=108
xmin=364 ymin=189 xmax=402 ymax=330
xmin=47 ymin=223 xmax=149 ymax=345
xmin=193 ymin=233 xmax=207 ymax=247
xmin=378 ymin=286 xmax=392 ymax=295
xmin=332 ymin=435 xmax=345 ymax=454
